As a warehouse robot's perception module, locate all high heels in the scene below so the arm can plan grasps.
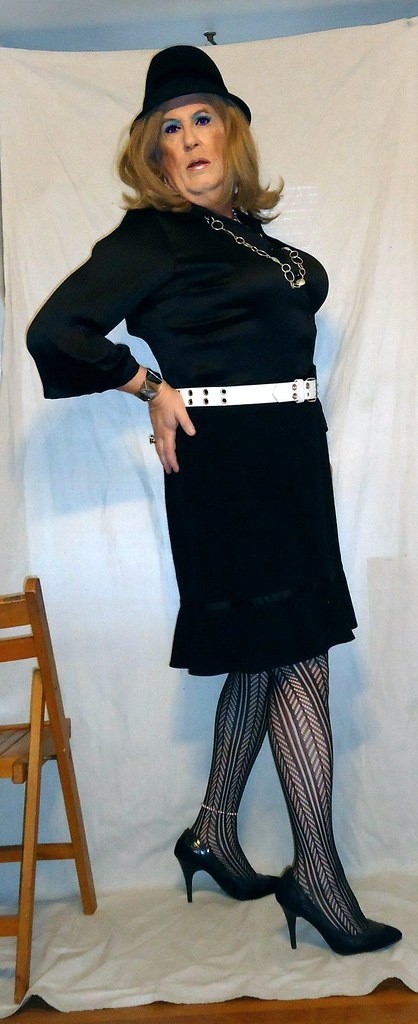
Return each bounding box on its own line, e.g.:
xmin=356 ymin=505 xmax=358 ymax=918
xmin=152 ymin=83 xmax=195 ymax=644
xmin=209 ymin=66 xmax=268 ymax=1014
xmin=174 ymin=828 xmax=281 ymax=903
xmin=275 ymin=865 xmax=402 ymax=956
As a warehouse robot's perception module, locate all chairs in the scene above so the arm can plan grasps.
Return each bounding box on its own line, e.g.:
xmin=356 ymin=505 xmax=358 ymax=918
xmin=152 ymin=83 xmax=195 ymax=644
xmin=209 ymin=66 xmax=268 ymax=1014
xmin=0 ymin=579 xmax=99 ymax=1004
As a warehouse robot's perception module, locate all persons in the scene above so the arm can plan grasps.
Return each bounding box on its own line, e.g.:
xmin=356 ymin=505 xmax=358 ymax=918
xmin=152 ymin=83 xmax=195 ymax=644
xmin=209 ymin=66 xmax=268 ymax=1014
xmin=26 ymin=46 xmax=402 ymax=958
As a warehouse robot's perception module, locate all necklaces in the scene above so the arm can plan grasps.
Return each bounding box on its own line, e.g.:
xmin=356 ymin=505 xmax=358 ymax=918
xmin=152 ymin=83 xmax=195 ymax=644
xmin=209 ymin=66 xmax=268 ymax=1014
xmin=205 ymin=215 xmax=306 ymax=290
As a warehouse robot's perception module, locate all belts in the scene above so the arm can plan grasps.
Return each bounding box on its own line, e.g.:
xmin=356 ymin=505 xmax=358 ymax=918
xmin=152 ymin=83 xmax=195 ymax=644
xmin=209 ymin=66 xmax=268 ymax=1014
xmin=174 ymin=378 xmax=317 ymax=407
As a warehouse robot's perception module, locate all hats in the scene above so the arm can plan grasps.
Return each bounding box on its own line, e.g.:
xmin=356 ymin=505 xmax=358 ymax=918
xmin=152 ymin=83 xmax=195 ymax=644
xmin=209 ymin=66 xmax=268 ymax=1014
xmin=129 ymin=45 xmax=252 ymax=137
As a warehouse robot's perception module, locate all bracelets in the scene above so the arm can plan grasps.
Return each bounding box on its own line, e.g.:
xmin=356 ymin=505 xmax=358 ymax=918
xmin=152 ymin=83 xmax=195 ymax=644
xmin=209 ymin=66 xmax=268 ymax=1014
xmin=135 ymin=370 xmax=161 ymax=401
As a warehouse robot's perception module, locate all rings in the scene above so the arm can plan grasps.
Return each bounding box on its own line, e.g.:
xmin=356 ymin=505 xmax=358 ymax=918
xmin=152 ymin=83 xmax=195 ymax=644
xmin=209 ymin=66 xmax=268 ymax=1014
xmin=150 ymin=435 xmax=155 ymax=443
